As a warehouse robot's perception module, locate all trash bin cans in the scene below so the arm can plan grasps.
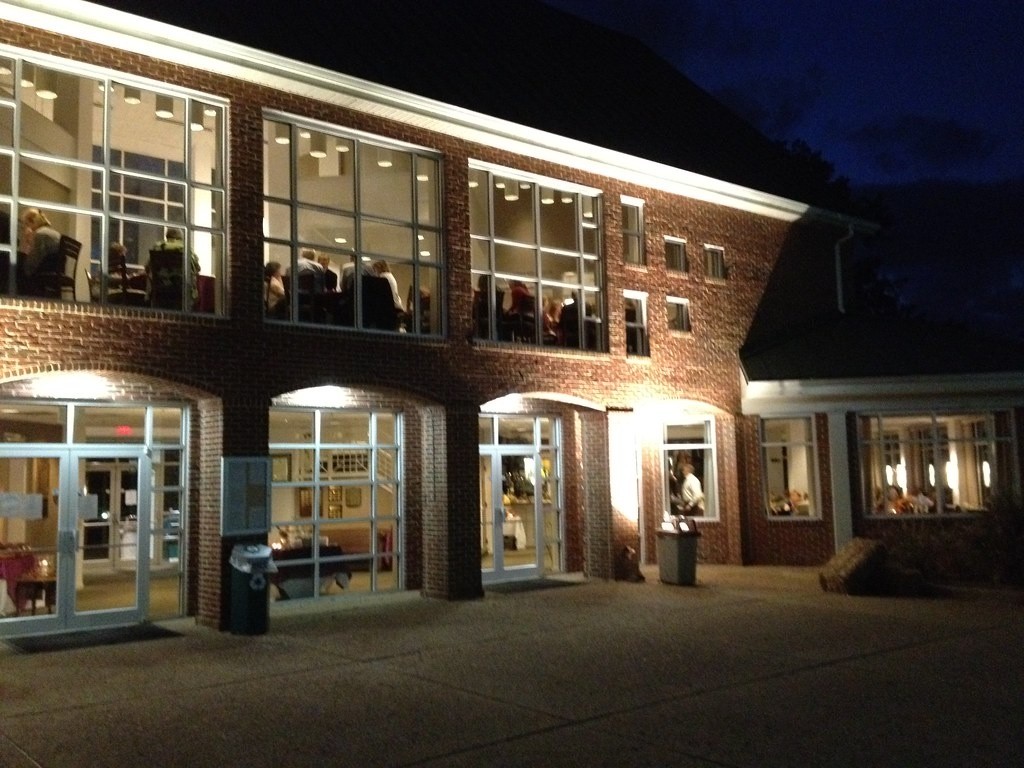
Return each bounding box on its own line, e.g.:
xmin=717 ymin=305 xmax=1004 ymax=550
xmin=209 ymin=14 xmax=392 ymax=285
xmin=229 ymin=543 xmax=278 ymax=636
xmin=654 ymin=519 xmax=702 ymax=586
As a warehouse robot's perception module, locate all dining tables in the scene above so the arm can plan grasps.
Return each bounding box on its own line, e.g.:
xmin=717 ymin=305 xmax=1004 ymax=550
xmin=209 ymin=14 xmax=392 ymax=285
xmin=322 ymin=290 xmax=345 ymax=327
xmin=127 ymin=272 xmax=215 ymax=314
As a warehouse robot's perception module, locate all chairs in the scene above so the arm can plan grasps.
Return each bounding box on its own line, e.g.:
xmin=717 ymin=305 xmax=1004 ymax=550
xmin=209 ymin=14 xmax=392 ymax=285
xmin=84 ymin=267 xmax=101 ymax=304
xmin=265 ymin=270 xmax=431 ymax=334
xmin=148 ymin=249 xmax=183 ymax=310
xmin=25 ymin=234 xmax=81 ymax=302
xmin=475 ymin=291 xmax=637 ymax=353
xmin=108 ymin=255 xmax=147 ymax=306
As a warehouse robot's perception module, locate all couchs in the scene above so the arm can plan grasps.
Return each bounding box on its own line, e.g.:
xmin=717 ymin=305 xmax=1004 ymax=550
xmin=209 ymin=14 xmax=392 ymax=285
xmin=306 ymin=527 xmax=382 ymax=572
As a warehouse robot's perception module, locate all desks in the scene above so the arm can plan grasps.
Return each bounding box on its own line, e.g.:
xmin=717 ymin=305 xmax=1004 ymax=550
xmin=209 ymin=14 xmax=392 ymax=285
xmin=16 ymin=576 xmax=56 ymax=616
xmin=272 ymin=542 xmax=353 ymax=599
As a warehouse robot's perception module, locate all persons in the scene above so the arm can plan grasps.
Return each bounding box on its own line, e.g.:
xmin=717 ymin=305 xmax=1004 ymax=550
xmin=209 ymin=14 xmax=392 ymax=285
xmin=668 ymin=458 xmax=679 ymax=500
xmin=265 ymin=245 xmax=599 ymax=349
xmin=15 ymin=208 xmax=65 ymax=296
xmin=680 ymin=464 xmax=704 ymax=517
xmin=769 ymin=482 xmax=960 ymax=520
xmin=142 ymin=225 xmax=200 ymax=309
xmin=92 ymin=240 xmax=133 ymax=297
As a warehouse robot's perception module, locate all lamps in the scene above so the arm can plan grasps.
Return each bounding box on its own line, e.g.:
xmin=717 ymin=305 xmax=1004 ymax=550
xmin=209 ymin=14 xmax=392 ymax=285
xmin=0 ymin=56 xmax=593 ymax=219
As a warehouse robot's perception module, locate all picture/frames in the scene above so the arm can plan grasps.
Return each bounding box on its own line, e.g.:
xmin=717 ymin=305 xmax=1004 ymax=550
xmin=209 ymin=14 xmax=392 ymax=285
xmin=271 ymin=453 xmax=291 ymax=483
xmin=298 ymin=485 xmax=362 ymax=519
